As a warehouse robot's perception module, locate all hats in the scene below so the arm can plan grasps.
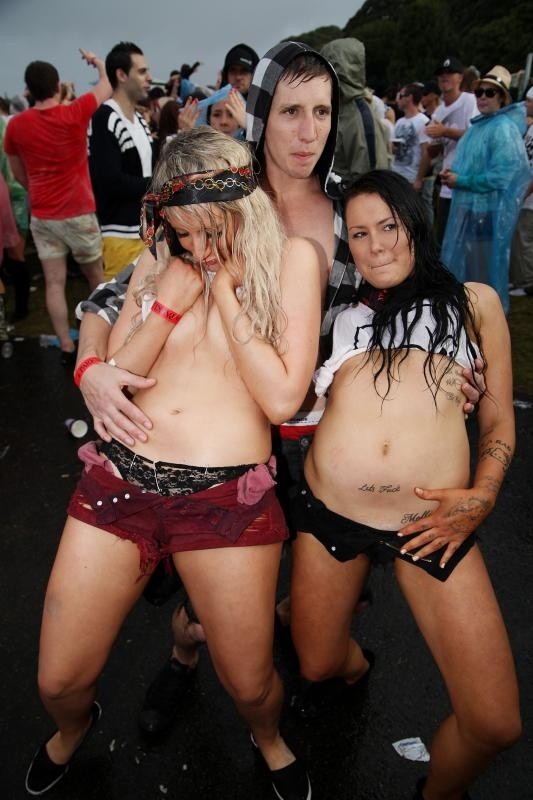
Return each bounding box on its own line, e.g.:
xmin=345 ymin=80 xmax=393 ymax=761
xmin=228 ymin=47 xmax=254 ymax=70
xmin=470 ymin=65 xmax=512 ymax=105
xmin=435 ymin=57 xmax=463 ymax=75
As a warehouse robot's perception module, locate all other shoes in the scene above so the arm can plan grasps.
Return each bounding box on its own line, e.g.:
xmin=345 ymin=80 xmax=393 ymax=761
xmin=509 ymin=288 xmax=527 ymax=296
xmin=25 ymin=701 xmax=102 ymax=796
xmin=250 ymin=731 xmax=312 ymax=800
xmin=288 ymin=649 xmax=376 ymax=726
xmin=138 ymin=653 xmax=199 ymax=733
xmin=61 ymin=348 xmax=78 ymax=367
xmin=412 ymin=777 xmax=471 ymax=800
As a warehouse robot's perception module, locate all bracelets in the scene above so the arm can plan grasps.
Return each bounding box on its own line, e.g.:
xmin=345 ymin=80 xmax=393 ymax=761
xmin=151 ymin=300 xmax=182 ymax=325
xmin=73 ymin=356 xmax=105 ymax=391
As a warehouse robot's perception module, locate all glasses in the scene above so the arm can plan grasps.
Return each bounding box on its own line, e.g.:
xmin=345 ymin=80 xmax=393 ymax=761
xmin=475 ymin=87 xmax=500 ymax=98
xmin=399 ymin=93 xmax=409 ymax=100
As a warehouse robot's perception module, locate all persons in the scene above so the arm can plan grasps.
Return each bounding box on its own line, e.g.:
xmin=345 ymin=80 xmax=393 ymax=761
xmin=0 ymin=38 xmax=533 ymax=360
xmin=26 ymin=128 xmax=323 ymax=800
xmin=71 ymin=39 xmax=488 ymax=774
xmin=288 ymin=172 xmax=519 ymax=800
